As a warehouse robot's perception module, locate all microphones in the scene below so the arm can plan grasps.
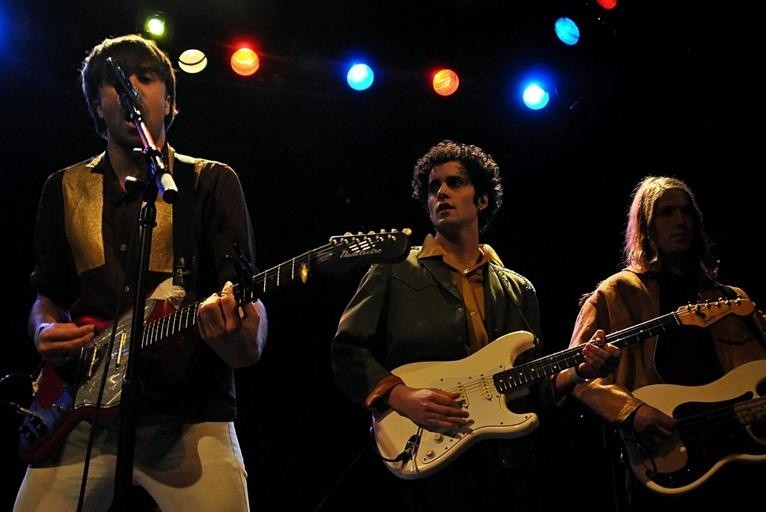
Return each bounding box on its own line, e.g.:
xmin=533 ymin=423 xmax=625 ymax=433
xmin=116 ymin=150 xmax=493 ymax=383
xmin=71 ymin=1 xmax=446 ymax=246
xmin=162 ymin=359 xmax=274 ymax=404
xmin=102 ymin=54 xmax=140 ymax=109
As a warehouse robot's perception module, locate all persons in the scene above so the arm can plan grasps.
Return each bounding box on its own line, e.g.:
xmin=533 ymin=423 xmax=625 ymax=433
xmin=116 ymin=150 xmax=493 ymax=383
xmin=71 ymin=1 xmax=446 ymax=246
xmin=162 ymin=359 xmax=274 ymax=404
xmin=328 ymin=140 xmax=623 ymax=512
xmin=567 ymin=174 xmax=766 ymax=511
xmin=11 ymin=33 xmax=269 ymax=512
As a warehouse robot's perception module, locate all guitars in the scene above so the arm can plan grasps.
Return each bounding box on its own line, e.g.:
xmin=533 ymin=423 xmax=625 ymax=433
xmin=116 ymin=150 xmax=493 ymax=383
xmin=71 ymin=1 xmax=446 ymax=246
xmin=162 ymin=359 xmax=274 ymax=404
xmin=372 ymin=296 xmax=757 ymax=480
xmin=17 ymin=227 xmax=413 ymax=464
xmin=619 ymin=359 xmax=766 ymax=494
